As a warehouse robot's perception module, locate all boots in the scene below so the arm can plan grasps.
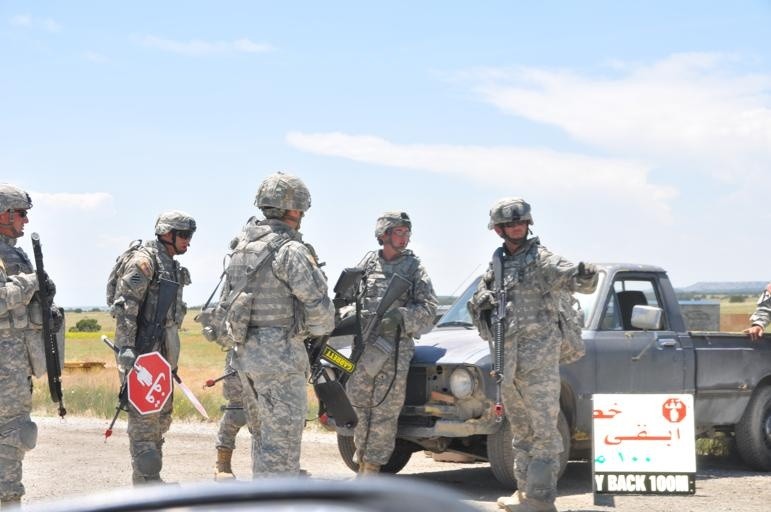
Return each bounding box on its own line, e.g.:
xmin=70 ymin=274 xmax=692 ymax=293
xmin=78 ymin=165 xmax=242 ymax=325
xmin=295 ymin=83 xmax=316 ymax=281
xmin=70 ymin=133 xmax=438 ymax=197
xmin=496 ymin=489 xmax=559 ymax=512
xmin=212 ymin=446 xmax=237 ymax=481
xmin=349 ymin=458 xmax=382 ymax=485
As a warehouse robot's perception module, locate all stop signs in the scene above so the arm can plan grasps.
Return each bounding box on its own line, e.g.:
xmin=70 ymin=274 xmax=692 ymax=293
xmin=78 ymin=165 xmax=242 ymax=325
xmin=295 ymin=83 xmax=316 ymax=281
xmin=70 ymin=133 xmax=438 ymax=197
xmin=121 ymin=353 xmax=175 ymax=416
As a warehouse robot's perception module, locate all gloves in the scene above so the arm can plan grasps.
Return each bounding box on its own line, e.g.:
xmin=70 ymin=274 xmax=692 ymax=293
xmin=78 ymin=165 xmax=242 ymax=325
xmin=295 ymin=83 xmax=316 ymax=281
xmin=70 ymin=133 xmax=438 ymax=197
xmin=380 ymin=307 xmax=403 ymax=332
xmin=475 ymin=290 xmax=498 ymax=311
xmin=117 ymin=346 xmax=137 ymax=369
xmin=5 ymin=268 xmax=56 ymax=303
xmin=572 ymin=261 xmax=598 ymax=280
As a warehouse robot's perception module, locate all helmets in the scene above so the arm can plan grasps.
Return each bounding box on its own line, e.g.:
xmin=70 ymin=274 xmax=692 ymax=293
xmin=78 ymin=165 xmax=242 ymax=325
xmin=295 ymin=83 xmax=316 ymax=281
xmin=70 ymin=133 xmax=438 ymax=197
xmin=154 ymin=208 xmax=197 ymax=236
xmin=374 ymin=210 xmax=412 ymax=238
xmin=253 ymin=169 xmax=312 ymax=212
xmin=0 ymin=182 xmax=34 ymax=214
xmin=487 ymin=197 xmax=534 ymax=230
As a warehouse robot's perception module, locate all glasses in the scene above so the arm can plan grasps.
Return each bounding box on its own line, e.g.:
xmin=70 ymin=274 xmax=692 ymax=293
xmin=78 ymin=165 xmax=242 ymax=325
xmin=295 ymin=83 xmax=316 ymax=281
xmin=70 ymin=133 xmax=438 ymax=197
xmin=7 ymin=209 xmax=28 ymax=217
xmin=502 ymin=219 xmax=526 ymax=227
xmin=391 ymin=230 xmax=412 ymax=236
xmin=176 ymin=231 xmax=193 ymax=239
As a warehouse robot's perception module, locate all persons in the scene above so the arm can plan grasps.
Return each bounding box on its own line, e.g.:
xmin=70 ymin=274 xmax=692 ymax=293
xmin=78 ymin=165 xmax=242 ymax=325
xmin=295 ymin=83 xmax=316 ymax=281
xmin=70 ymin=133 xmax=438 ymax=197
xmin=0 ymin=184 xmax=63 ymax=512
xmin=345 ymin=210 xmax=437 ymax=476
xmin=742 ymin=280 xmax=771 ymax=342
xmin=213 ymin=349 xmax=247 ymax=479
xmin=228 ymin=170 xmax=336 ymax=476
xmin=114 ymin=210 xmax=196 ymax=487
xmin=477 ymin=197 xmax=597 ymax=512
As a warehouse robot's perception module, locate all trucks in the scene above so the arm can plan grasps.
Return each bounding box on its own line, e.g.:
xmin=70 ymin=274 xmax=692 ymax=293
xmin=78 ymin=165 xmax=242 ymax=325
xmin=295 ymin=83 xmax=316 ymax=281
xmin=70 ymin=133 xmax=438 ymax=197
xmin=309 ymin=252 xmax=771 ymax=482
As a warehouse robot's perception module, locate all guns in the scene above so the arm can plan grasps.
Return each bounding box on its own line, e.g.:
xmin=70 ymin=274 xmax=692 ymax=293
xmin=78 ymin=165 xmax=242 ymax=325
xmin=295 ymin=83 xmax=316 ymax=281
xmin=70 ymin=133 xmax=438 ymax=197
xmin=31 ymin=232 xmax=65 ymax=416
xmin=304 ymin=267 xmax=411 ymax=425
xmin=492 ymin=247 xmax=506 ymax=422
xmin=104 ymin=279 xmax=180 ymax=435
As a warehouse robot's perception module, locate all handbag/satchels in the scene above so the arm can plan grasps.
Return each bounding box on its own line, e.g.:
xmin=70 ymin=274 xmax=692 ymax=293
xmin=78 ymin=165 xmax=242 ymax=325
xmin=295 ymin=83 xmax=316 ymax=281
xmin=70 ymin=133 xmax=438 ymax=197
xmin=26 ymin=307 xmax=67 ymax=379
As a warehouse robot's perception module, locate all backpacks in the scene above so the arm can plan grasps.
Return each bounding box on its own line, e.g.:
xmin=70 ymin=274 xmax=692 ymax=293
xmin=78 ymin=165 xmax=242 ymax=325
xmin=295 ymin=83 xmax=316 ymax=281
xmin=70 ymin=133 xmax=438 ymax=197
xmin=193 ymin=231 xmax=289 ymax=347
xmin=106 ymin=239 xmax=143 ymax=307
xmin=559 ymin=291 xmax=586 ymax=366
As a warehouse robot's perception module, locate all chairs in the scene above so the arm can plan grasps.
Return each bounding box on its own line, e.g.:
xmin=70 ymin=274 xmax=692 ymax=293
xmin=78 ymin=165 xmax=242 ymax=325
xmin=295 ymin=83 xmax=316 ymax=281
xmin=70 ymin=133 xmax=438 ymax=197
xmin=611 ymin=290 xmax=653 ymax=329
